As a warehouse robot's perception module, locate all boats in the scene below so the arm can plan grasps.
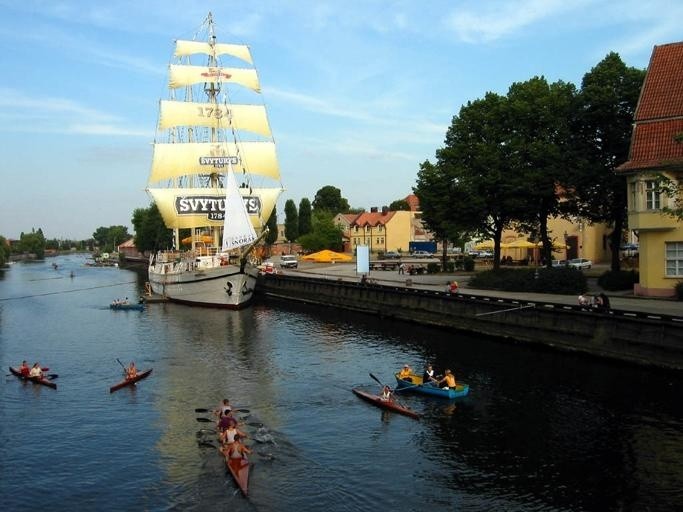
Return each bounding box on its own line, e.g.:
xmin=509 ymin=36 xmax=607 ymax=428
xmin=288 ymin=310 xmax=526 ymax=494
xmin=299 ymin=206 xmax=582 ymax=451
xmin=352 ymin=388 xmax=419 ymax=421
xmin=110 ymin=368 xmax=153 ymax=393
xmin=218 ymin=400 xmax=250 ymax=494
xmin=109 ymin=304 xmax=144 ymax=311
xmin=84 ymin=262 xmax=118 ymax=267
xmin=9 ymin=366 xmax=57 ymax=390
xmin=394 ymin=372 xmax=469 ymax=398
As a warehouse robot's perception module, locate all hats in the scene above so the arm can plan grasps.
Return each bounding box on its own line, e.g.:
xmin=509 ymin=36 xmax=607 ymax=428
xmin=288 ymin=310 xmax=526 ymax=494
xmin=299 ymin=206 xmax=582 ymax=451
xmin=225 ymin=409 xmax=232 ymax=415
xmin=403 ymin=364 xmax=410 ymax=368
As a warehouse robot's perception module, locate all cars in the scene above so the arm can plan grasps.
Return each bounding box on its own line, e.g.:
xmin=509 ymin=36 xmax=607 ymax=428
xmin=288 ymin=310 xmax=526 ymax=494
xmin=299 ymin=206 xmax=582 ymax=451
xmin=280 ymin=255 xmax=298 ymax=268
xmin=412 ymin=250 xmax=433 ymax=259
xmin=542 ymin=259 xmax=592 ymax=270
xmin=470 ymin=251 xmax=493 ymax=258
xmin=384 ymin=252 xmax=401 ymax=258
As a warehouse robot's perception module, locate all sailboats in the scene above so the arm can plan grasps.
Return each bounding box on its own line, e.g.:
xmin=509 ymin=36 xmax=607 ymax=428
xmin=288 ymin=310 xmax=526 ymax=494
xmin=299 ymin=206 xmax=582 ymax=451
xmin=148 ymin=13 xmax=284 ymax=306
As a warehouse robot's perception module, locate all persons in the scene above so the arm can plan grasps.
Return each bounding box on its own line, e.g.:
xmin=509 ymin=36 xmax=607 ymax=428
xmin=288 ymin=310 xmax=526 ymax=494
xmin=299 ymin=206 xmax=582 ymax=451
xmin=439 ymin=368 xmax=457 ymax=390
xmin=19 ymin=358 xmax=30 ymax=379
xmin=398 ymin=363 xmax=416 ymax=382
xmin=445 ymin=279 xmax=462 ymax=295
xmin=124 ymin=359 xmax=137 ymax=382
xmin=377 ymin=384 xmax=399 ymax=404
xmin=29 ymin=362 xmax=44 ymax=380
xmin=422 ymin=362 xmax=435 ymax=384
xmin=397 ymin=262 xmax=426 ymax=276
xmin=112 ymin=295 xmax=129 ymax=307
xmin=577 ymin=290 xmax=611 ymax=309
xmin=211 ymin=398 xmax=254 ymax=461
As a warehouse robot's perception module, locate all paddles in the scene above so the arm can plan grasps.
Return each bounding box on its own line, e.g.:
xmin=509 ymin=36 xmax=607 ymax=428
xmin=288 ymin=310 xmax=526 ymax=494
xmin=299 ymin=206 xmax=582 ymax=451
xmin=199 ymin=429 xmax=263 ymax=444
xmin=196 ymin=417 xmax=262 ymax=427
xmin=197 ymin=442 xmax=275 ymax=460
xmin=368 ymin=372 xmax=405 ymax=410
xmin=194 ymin=408 xmax=249 ymax=413
xmin=5 ymin=371 xmax=19 ymax=376
xmin=116 ymin=357 xmax=130 ymax=378
